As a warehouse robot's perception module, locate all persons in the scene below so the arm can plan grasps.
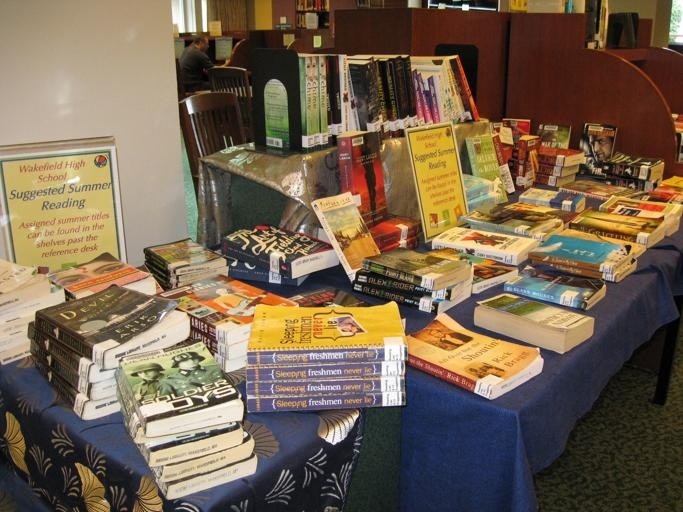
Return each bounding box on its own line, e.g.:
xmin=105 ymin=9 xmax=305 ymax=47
xmin=179 ymin=30 xmax=221 ymax=90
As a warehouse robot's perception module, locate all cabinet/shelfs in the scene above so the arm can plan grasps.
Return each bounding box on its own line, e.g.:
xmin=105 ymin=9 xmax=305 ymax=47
xmin=293 ymin=0 xmax=359 ymax=39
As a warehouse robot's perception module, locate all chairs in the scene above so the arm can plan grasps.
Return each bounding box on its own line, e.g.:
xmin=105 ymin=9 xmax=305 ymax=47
xmin=176 ymin=57 xmax=256 ymax=197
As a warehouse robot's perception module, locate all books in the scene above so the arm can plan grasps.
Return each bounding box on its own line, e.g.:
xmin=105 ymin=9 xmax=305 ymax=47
xmin=1 ymin=117 xmax=682 ymax=500
xmin=500 ymin=1 xmax=641 ymax=50
xmin=262 ymin=54 xmax=481 ymax=154
xmin=295 ymin=0 xmax=330 ymax=29
